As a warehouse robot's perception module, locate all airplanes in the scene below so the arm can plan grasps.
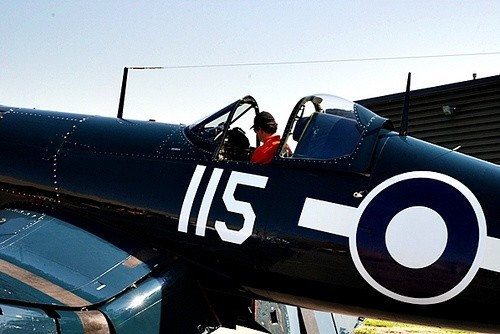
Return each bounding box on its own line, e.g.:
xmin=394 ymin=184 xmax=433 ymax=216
xmin=0 ymin=93 xmax=500 ymax=334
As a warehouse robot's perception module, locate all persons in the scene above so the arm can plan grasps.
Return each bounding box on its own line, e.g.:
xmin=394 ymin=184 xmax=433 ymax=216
xmin=248 ymin=111 xmax=292 ymax=164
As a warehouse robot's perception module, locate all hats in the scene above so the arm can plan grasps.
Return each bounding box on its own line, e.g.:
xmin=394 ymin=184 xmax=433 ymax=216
xmin=250 ymin=111 xmax=278 ymax=130
